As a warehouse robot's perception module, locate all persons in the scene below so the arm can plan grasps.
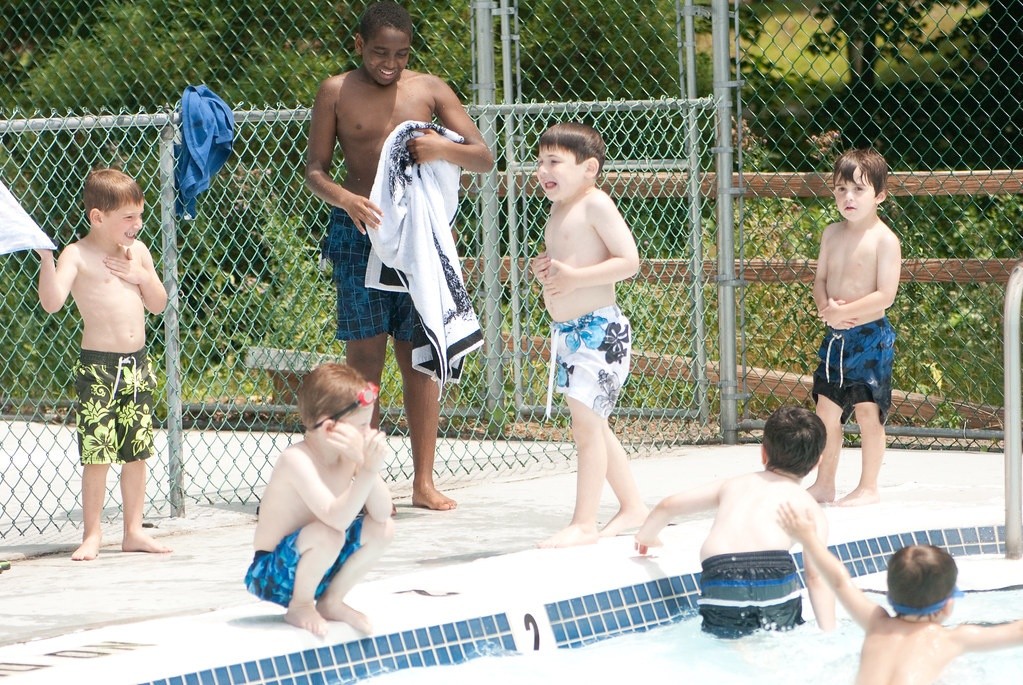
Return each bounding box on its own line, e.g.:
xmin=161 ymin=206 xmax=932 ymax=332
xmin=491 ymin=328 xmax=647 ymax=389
xmin=806 ymin=149 xmax=901 ymax=503
xmin=533 ymin=123 xmax=648 ymax=550
xmin=246 ymin=364 xmax=394 ymax=635
xmin=308 ymin=0 xmax=493 ymax=516
xmin=634 ymin=407 xmax=835 ymax=639
xmin=775 ymin=500 xmax=1023 ymax=685
xmin=36 ymin=169 xmax=167 ymax=560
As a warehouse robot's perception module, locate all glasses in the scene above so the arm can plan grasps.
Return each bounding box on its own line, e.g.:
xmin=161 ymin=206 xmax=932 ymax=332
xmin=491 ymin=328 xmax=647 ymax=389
xmin=314 ymin=382 xmax=380 ymax=427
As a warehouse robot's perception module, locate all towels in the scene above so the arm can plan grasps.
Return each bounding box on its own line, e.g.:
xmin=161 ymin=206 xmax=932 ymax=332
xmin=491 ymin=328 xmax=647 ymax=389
xmin=0 ymin=180 xmax=58 ymax=256
xmin=363 ymin=120 xmax=485 ymax=402
xmin=174 ymin=84 xmax=236 ymax=221
